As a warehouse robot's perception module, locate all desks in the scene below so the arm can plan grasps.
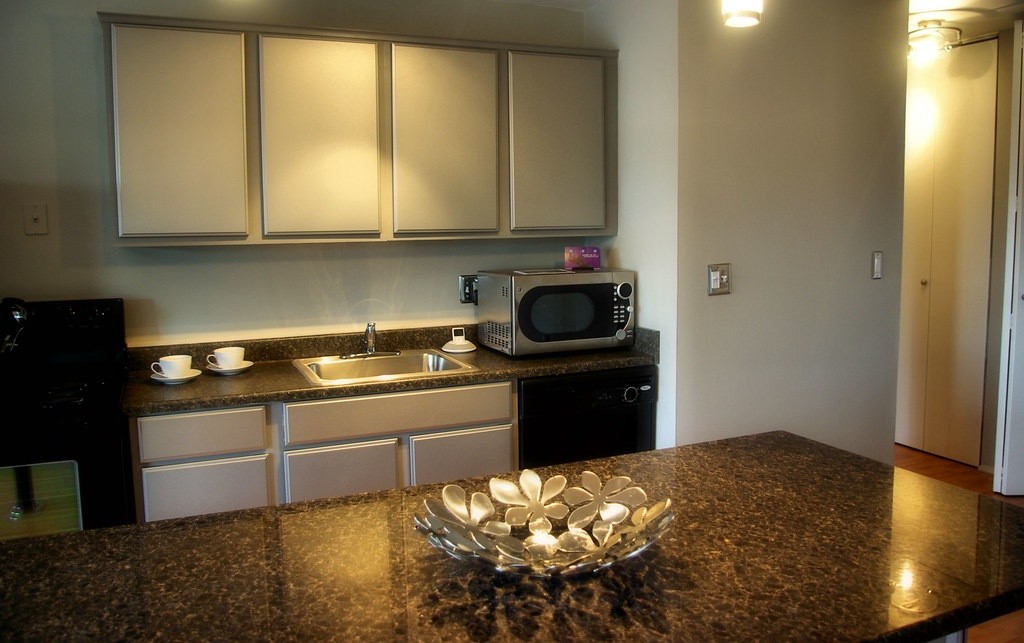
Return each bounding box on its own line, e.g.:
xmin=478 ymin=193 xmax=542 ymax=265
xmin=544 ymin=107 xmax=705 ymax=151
xmin=0 ymin=430 xmax=1024 ymax=643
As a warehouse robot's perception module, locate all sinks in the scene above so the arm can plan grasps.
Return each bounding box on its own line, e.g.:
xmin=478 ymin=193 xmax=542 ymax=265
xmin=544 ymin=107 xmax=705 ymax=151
xmin=308 ymin=350 xmax=481 ymax=386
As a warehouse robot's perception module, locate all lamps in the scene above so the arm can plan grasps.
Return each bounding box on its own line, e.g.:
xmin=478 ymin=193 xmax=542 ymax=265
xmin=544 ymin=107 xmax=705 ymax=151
xmin=720 ymin=0 xmax=762 ymax=27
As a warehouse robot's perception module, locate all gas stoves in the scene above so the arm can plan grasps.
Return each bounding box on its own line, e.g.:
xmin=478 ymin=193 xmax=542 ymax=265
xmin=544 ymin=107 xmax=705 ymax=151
xmin=0 ymin=296 xmax=125 ymax=418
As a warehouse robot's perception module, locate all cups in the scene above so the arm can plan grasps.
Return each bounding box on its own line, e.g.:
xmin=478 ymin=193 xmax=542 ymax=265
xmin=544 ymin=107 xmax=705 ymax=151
xmin=151 ymin=355 xmax=192 ymax=378
xmin=207 ymin=347 xmax=245 ymax=369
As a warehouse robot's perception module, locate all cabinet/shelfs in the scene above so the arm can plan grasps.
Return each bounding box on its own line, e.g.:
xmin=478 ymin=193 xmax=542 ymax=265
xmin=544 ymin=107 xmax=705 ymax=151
xmin=108 ymin=20 xmax=617 ymax=247
xmin=129 ymin=380 xmax=518 ymax=524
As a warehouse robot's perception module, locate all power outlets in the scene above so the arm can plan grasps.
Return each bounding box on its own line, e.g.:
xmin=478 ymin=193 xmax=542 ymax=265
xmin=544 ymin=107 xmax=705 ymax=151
xmin=459 ymin=275 xmax=478 ymax=303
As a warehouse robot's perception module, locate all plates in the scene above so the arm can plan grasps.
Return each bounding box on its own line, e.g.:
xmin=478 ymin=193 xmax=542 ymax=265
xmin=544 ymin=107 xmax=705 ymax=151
xmin=151 ymin=369 xmax=202 ymax=384
xmin=206 ymin=361 xmax=254 ymax=375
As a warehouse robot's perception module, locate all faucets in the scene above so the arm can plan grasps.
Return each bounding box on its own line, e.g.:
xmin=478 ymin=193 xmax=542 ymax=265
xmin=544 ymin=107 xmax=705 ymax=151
xmin=364 ymin=322 xmax=377 ymax=354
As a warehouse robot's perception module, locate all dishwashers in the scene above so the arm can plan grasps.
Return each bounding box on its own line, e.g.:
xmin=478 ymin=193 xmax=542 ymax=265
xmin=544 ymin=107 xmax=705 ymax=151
xmin=516 ymin=365 xmax=659 ymax=470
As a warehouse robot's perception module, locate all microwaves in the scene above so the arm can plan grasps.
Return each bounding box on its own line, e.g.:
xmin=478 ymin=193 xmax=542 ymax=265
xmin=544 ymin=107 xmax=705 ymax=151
xmin=477 ymin=267 xmax=635 ymax=357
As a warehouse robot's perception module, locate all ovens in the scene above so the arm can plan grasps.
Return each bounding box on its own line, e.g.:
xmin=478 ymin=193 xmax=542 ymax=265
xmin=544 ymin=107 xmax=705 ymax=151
xmin=0 ymin=410 xmax=138 ymax=541
